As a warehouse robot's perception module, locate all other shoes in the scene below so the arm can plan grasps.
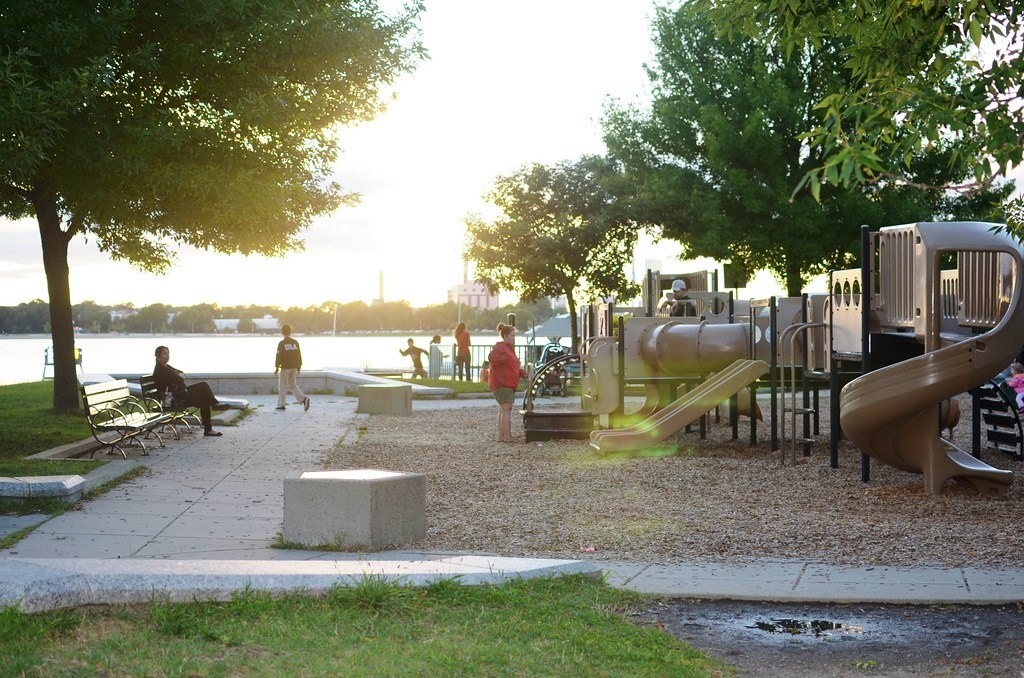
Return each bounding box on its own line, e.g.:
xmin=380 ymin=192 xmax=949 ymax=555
xmin=276 ymin=407 xmax=285 ymax=411
xmin=498 ymin=438 xmax=518 ymax=443
xmin=212 ymin=404 xmax=230 ymax=411
xmin=204 ymin=429 xmax=223 ymax=436
xmin=304 ymin=396 xmax=309 ymax=411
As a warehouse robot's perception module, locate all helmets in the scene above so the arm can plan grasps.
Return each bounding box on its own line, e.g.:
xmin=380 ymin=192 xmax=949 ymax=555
xmin=672 ymin=279 xmax=686 ymax=291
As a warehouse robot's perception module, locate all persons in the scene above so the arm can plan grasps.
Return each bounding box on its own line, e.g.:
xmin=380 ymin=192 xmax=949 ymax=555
xmin=275 ymin=325 xmax=309 ymax=411
xmin=1007 ymin=362 xmax=1024 ymax=413
xmin=153 ymin=346 xmax=230 ymax=436
xmin=430 ymin=335 xmax=443 ymax=380
xmin=480 ymin=361 xmax=490 ymax=383
xmin=488 ymin=322 xmax=528 ymax=443
xmin=399 ymin=339 xmax=429 ymax=379
xmin=454 ymin=322 xmax=471 ymax=381
xmin=660 ymin=279 xmax=698 ymax=317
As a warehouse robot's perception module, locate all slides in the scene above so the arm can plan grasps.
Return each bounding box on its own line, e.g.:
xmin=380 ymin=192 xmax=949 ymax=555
xmin=838 ymin=314 xmax=1023 ymax=497
xmin=588 ymin=358 xmax=771 ymax=456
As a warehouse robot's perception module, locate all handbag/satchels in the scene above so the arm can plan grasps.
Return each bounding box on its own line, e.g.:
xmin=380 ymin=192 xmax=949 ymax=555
xmin=455 ymin=357 xmax=461 ymax=365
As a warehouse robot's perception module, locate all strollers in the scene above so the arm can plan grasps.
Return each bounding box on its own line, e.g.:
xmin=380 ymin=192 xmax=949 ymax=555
xmin=534 ymin=342 xmax=567 ymax=397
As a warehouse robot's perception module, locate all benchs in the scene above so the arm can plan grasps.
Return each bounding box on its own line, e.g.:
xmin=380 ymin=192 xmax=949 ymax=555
xmin=140 ymin=374 xmax=203 ymax=440
xmin=81 ymin=378 xmax=172 ymax=461
